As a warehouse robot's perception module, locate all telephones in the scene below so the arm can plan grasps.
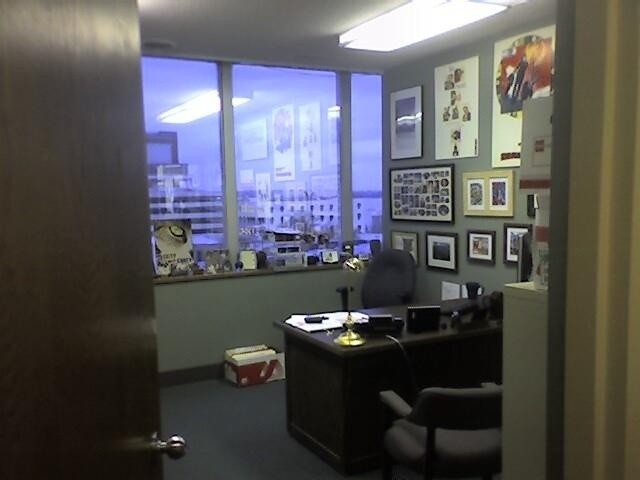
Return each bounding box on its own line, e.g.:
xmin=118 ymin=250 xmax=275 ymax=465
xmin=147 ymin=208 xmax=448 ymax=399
xmin=353 ymin=315 xmax=404 ymax=336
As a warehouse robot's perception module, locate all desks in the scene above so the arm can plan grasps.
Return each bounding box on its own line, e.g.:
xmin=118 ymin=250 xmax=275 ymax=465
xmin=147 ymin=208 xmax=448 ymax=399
xmin=273 ymin=298 xmax=503 ymax=477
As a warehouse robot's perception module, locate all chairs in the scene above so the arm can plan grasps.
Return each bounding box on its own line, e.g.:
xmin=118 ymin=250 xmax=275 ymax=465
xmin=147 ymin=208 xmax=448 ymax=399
xmin=361 ymin=248 xmax=416 ymax=311
xmin=379 ymin=381 xmax=502 ymax=480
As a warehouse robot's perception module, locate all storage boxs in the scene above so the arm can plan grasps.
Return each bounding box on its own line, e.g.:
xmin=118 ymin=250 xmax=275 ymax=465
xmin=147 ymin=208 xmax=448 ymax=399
xmin=224 ymin=344 xmax=276 ymax=388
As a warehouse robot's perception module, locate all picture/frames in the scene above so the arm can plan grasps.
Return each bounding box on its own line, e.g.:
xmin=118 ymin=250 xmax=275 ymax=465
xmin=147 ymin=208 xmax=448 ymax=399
xmin=390 ymin=230 xmax=419 ymax=269
xmin=502 ymin=222 xmax=532 ymax=265
xmin=462 ymin=171 xmax=487 ymax=217
xmin=486 ymin=170 xmax=515 ymax=218
xmin=425 ymin=230 xmax=459 ymax=272
xmin=389 ymin=84 xmax=424 ymax=161
xmin=388 ymin=163 xmax=455 ymax=224
xmin=466 ymin=229 xmax=496 ymax=265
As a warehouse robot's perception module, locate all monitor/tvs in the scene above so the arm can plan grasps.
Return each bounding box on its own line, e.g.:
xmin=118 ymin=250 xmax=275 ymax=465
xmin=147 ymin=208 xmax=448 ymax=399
xmin=517 ymin=232 xmax=532 ymax=282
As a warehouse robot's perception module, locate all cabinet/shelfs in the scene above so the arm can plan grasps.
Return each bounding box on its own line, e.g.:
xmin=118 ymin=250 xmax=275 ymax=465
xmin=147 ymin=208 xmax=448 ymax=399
xmin=503 ymin=282 xmax=548 ymax=480
